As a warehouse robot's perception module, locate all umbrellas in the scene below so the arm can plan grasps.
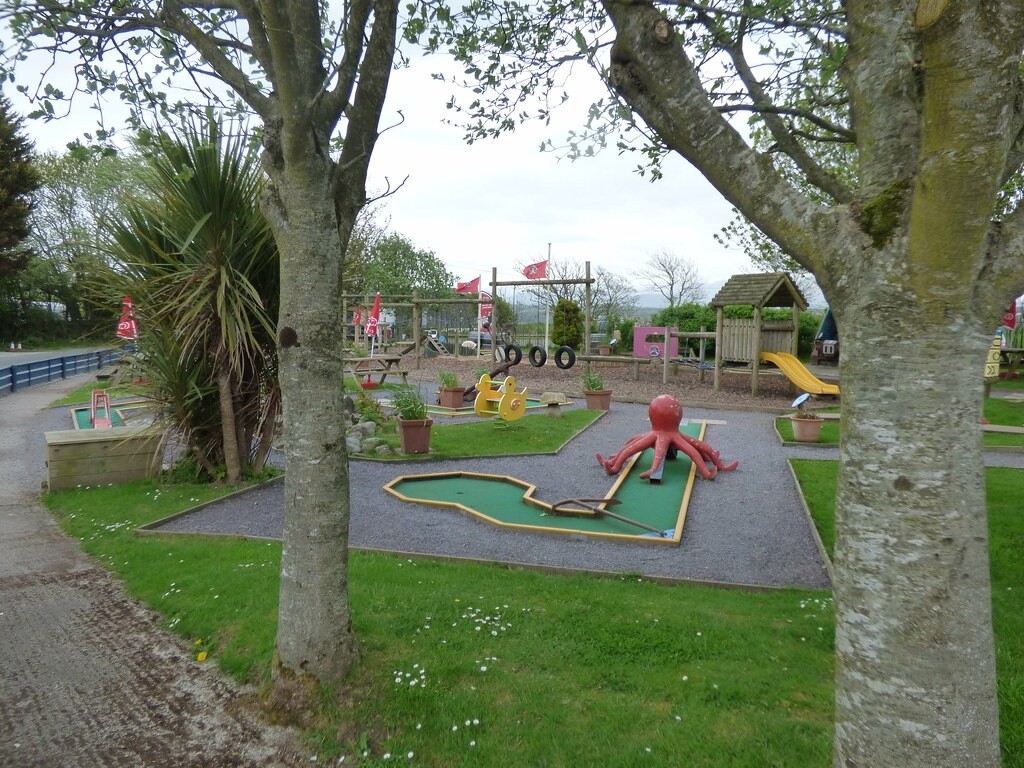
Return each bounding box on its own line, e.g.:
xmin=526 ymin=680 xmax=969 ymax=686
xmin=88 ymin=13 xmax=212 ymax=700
xmin=117 ymin=296 xmax=140 ymax=339
xmin=364 ymin=292 xmax=380 ymax=358
xmin=353 ymin=305 xmax=362 ymax=345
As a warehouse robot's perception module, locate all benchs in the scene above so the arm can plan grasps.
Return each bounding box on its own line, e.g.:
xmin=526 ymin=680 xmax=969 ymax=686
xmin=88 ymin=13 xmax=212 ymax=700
xmin=95 ymin=366 xmax=120 ymax=379
xmin=341 ymin=368 xmax=387 ymax=372
xmin=353 ymin=371 xmax=410 ymax=385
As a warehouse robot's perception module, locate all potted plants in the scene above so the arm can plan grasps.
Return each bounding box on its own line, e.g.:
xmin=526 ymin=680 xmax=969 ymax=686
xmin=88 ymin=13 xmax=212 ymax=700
xmin=790 ymin=405 xmax=824 ymax=442
xmin=439 ymin=369 xmax=466 ymax=408
xmin=579 ymin=365 xmax=613 ymax=410
xmin=598 ymin=338 xmax=610 ymax=356
xmin=388 ymin=375 xmax=434 ymax=454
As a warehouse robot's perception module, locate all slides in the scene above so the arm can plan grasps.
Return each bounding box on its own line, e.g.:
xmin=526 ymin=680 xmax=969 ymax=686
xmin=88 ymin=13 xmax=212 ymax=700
xmin=759 ymin=351 xmax=840 ymax=394
xmin=396 ymin=334 xmax=427 ymax=357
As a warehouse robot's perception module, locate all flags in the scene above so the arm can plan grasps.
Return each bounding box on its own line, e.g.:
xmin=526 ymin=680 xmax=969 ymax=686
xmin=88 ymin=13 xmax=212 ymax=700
xmin=523 ymin=259 xmax=548 ymax=279
xmin=456 ymin=276 xmax=480 ymax=292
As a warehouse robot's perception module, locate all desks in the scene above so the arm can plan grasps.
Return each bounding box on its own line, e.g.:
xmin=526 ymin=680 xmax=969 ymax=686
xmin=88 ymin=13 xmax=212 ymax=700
xmin=117 ymin=353 xmax=151 ymax=382
xmin=342 ymin=356 xmax=403 ymax=372
